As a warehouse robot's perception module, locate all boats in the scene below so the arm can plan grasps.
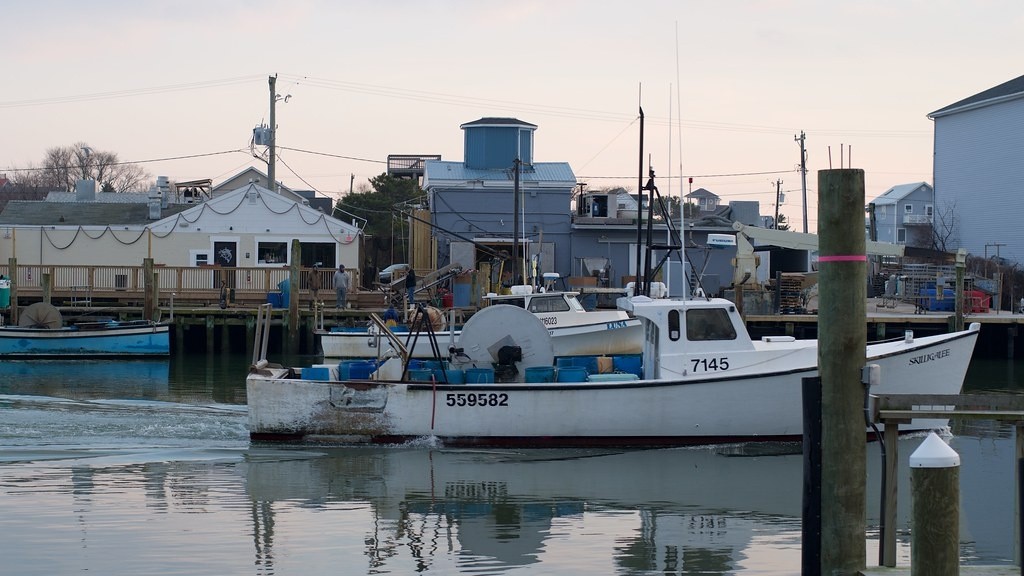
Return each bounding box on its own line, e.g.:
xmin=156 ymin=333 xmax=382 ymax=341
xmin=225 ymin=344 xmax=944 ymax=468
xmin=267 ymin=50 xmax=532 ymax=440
xmin=245 ymin=19 xmax=981 ymax=449
xmin=0 ymin=320 xmax=174 ymax=357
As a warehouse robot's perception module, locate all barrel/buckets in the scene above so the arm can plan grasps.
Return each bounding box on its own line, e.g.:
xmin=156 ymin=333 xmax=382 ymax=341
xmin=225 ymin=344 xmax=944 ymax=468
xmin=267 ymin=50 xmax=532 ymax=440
xmin=301 ymin=355 xmax=641 ymax=384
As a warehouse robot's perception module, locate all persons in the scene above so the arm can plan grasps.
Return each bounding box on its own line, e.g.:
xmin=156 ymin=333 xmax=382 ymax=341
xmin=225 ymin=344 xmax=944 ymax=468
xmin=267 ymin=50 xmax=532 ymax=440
xmin=865 ymin=272 xmax=877 ymax=298
xmin=889 ymin=272 xmax=899 ymax=295
xmin=307 ymin=264 xmax=322 ymax=312
xmin=332 ymin=264 xmax=352 ymax=312
xmin=362 ymin=256 xmax=377 ymax=292
xmin=382 ymin=304 xmax=400 ymax=328
xmin=404 ymin=265 xmax=416 ymax=310
